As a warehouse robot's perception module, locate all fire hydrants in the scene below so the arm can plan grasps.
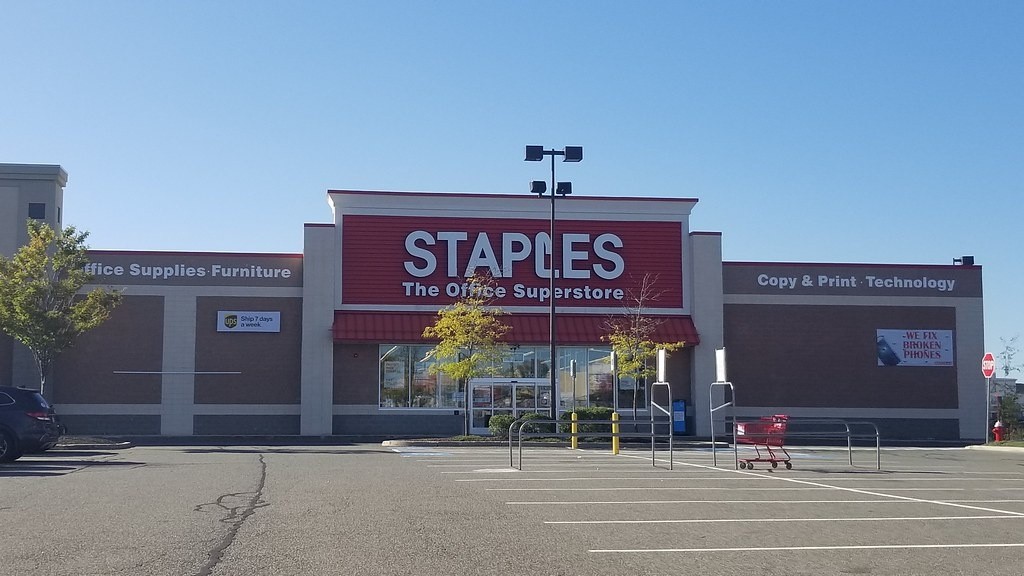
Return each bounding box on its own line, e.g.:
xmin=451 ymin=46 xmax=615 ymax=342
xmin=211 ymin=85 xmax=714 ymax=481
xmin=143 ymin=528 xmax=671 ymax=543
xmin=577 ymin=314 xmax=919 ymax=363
xmin=992 ymin=420 xmax=1006 ymax=441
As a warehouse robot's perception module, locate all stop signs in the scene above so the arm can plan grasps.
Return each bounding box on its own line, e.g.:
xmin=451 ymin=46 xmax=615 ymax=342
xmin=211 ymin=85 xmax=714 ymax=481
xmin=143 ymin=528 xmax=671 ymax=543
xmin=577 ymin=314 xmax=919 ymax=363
xmin=981 ymin=353 xmax=995 ymax=378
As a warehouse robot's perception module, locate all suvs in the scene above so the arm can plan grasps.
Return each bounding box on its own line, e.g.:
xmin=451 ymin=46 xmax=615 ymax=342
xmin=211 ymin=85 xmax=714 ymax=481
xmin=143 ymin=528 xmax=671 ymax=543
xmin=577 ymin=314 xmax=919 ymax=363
xmin=0 ymin=385 xmax=62 ymax=463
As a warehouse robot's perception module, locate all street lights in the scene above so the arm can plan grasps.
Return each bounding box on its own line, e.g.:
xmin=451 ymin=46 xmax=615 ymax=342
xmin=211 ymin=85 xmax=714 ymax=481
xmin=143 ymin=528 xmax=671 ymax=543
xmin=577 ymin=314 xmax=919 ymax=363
xmin=525 ymin=146 xmax=583 ymax=433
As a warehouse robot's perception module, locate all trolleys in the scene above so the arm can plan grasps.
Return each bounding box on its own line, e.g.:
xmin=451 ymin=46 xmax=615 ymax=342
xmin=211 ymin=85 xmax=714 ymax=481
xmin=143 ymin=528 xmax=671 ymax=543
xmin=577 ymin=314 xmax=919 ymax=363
xmin=735 ymin=414 xmax=794 ymax=470
